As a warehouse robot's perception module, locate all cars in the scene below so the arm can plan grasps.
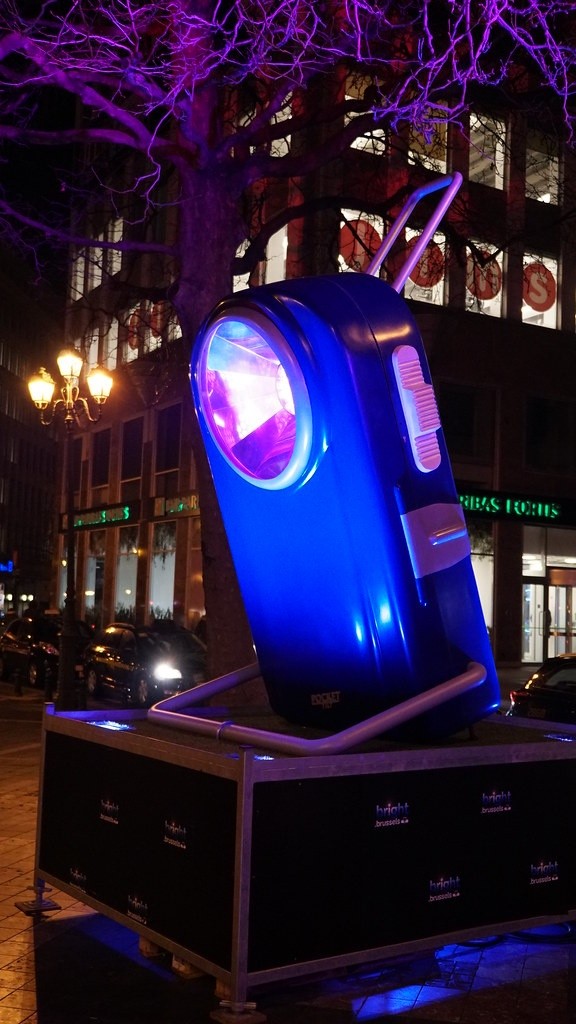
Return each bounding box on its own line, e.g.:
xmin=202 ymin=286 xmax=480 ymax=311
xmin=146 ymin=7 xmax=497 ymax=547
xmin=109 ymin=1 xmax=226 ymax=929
xmin=0 ymin=615 xmax=210 ymax=711
xmin=505 ymin=658 xmax=576 ymax=725
